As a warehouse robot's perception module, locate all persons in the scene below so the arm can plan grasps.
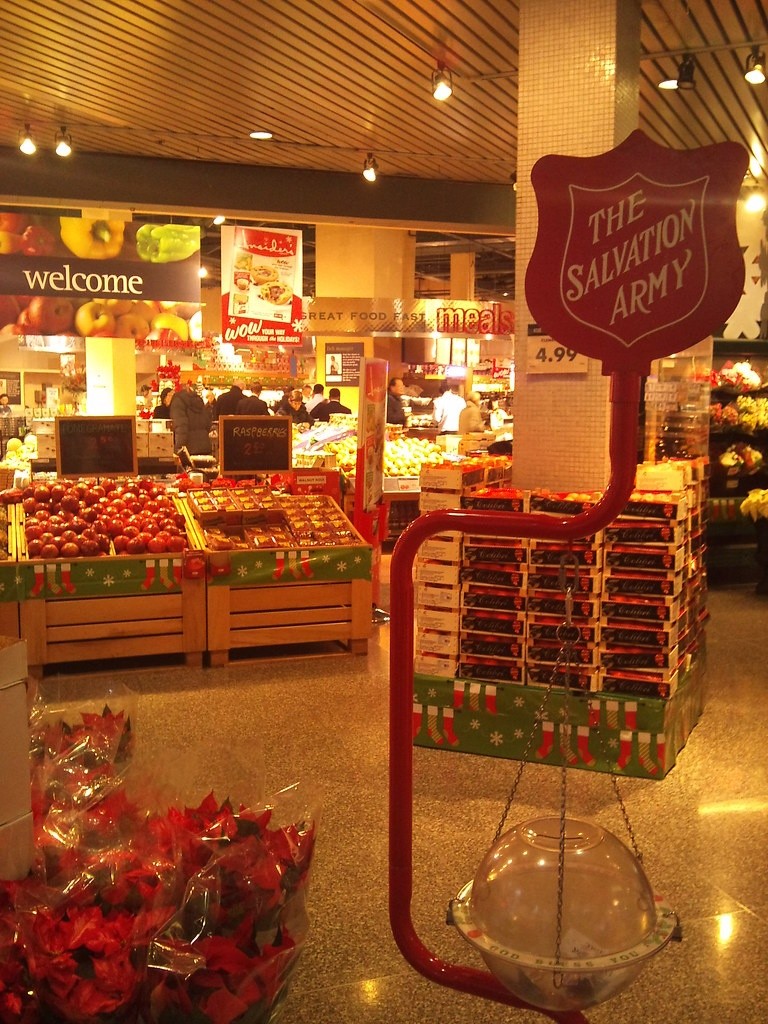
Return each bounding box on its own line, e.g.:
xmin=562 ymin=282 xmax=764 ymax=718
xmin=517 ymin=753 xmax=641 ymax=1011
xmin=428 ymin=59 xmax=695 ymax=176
xmin=329 ymin=356 xmax=338 ymax=376
xmin=0 ymin=393 xmax=12 ymax=416
xmin=141 ymin=380 xmax=353 ymax=473
xmin=431 ymin=384 xmax=516 ymax=432
xmin=386 ymin=377 xmax=407 ymax=425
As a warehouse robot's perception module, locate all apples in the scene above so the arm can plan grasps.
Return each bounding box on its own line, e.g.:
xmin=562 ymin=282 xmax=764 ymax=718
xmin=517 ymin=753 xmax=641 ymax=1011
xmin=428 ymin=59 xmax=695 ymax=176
xmin=384 ymin=438 xmax=443 ymax=476
xmin=1 ymin=294 xmax=201 ymax=343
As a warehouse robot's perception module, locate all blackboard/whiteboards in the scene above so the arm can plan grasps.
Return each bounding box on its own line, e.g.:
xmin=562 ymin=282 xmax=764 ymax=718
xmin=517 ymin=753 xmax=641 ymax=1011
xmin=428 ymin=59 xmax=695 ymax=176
xmin=218 ymin=416 xmax=291 ymax=474
xmin=54 ymin=416 xmax=137 ymax=480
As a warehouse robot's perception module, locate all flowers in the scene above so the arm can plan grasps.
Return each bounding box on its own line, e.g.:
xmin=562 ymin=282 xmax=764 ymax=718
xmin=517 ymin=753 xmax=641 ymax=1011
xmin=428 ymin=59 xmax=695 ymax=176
xmin=692 ymin=358 xmax=768 ymax=522
xmin=0 ymin=704 xmax=315 ymax=1024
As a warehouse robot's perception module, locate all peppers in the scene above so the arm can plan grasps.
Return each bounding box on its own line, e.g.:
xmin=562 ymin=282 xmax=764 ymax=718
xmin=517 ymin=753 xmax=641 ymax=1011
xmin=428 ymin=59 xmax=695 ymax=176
xmin=60 ymin=217 xmax=124 ymax=259
xmin=137 ymin=225 xmax=200 ymax=263
xmin=0 ymin=231 xmax=23 ymax=254
xmin=21 ymin=226 xmax=55 ymax=256
xmin=0 ymin=212 xmax=32 ymax=234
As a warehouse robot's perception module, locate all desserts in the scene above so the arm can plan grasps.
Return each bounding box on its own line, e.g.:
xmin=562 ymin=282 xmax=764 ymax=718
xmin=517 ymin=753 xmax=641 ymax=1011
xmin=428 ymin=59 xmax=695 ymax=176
xmin=237 ymin=279 xmax=248 ymax=289
xmin=236 ymin=255 xmax=252 ymax=270
xmin=251 ymin=266 xmax=278 ymax=284
xmin=261 ymin=282 xmax=292 ymax=305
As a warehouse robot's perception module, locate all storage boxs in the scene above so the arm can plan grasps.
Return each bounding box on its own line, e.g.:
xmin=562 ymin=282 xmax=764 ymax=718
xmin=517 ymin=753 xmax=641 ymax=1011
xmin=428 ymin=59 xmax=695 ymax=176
xmin=0 ymin=635 xmax=36 ymax=881
xmin=412 ymin=460 xmax=711 ymax=700
xmin=135 ymin=419 xmax=174 ymax=458
xmin=35 ymin=420 xmax=57 ymax=460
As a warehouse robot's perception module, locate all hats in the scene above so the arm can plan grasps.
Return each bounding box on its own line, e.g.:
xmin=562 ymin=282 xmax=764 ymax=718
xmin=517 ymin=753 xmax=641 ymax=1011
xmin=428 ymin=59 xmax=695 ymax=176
xmin=141 ymin=385 xmax=152 ymax=392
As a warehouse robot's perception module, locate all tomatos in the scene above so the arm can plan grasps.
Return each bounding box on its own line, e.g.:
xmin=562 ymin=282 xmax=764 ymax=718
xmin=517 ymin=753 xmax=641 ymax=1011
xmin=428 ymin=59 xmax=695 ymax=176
xmin=24 ymin=478 xmax=186 ymax=556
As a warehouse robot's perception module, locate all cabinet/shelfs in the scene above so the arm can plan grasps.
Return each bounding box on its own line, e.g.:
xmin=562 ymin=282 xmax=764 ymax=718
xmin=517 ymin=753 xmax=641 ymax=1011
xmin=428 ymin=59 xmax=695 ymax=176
xmin=0 ymin=468 xmax=419 ymax=682
xmin=0 ymin=369 xmax=89 ymax=457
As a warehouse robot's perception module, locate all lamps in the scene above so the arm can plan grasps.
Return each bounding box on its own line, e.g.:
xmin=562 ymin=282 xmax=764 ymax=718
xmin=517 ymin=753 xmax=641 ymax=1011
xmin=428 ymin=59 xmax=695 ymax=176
xmin=432 ymin=61 xmax=452 ymax=101
xmin=54 ymin=126 xmax=72 ymax=157
xmin=17 ymin=124 xmax=36 ymax=154
xmin=677 ymin=55 xmax=697 ymax=89
xmin=744 ymin=45 xmax=766 ymax=85
xmin=363 ymin=153 xmax=378 ymax=182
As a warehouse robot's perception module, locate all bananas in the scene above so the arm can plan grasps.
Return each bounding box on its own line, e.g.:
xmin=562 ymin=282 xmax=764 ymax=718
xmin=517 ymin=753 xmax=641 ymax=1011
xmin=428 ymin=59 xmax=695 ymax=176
xmin=737 ymin=396 xmax=768 ymax=432
